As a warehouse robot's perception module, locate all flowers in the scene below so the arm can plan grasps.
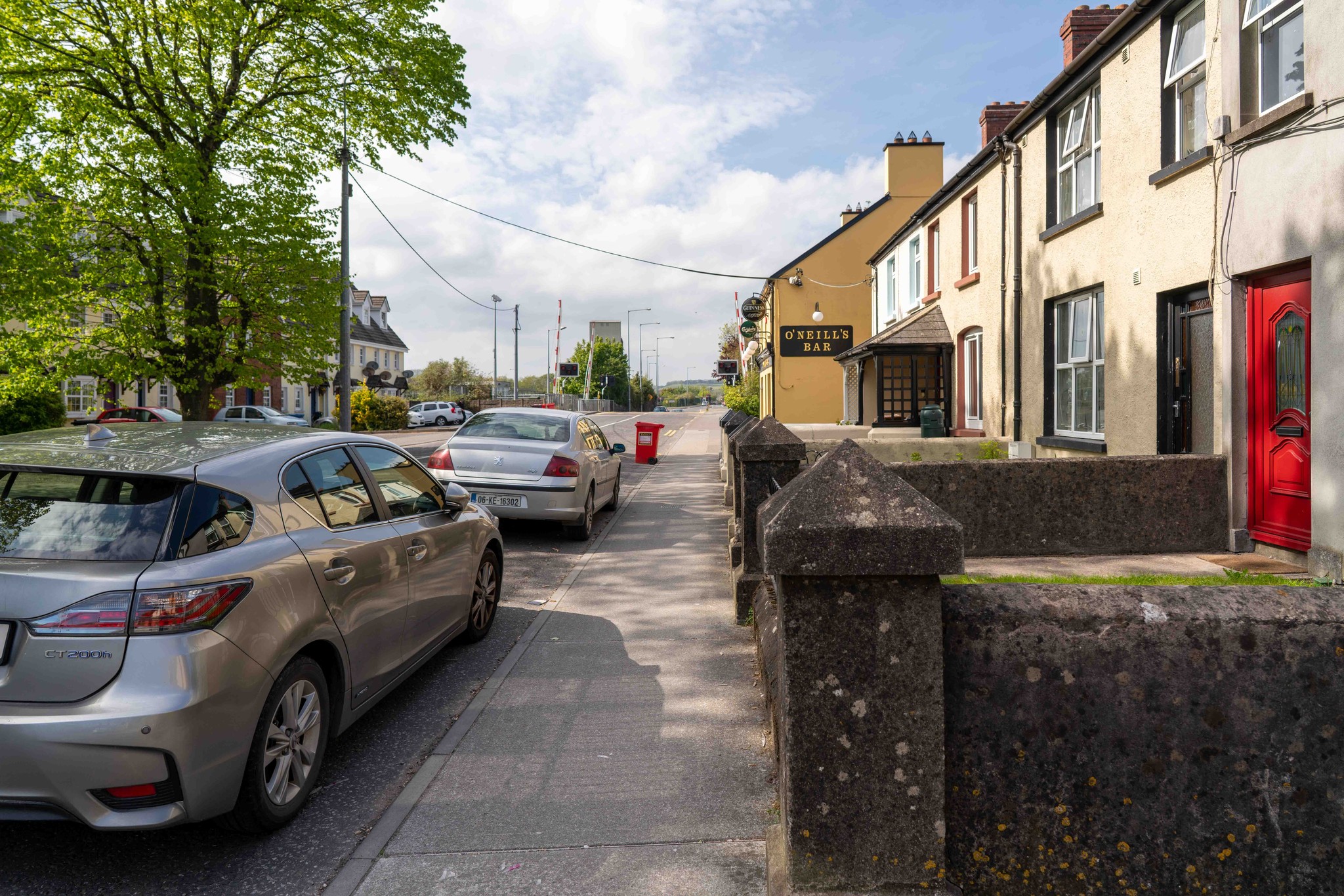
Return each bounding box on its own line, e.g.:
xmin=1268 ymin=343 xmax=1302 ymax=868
xmin=319 ymin=387 xmax=326 ymax=391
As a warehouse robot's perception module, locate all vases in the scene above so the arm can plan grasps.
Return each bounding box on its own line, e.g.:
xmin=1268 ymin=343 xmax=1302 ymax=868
xmin=320 ymin=391 xmax=326 ymax=396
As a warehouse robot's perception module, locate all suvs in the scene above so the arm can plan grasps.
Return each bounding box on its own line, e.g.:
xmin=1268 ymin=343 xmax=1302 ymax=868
xmin=407 ymin=401 xmax=464 ymax=426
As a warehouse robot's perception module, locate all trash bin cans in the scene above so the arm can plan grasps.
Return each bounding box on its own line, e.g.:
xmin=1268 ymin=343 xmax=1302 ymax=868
xmin=542 ymin=403 xmax=555 ymax=409
xmin=531 ymin=404 xmax=542 ymax=408
xmin=634 ymin=421 xmax=665 ymax=464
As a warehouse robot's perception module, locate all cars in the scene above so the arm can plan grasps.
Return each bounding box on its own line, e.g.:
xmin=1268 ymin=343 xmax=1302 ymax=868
xmin=653 ymin=406 xmax=670 ymax=412
xmin=702 ymin=401 xmax=708 ymax=406
xmin=210 ymin=404 xmax=311 ymax=429
xmin=671 ymin=409 xmax=684 ymax=413
xmin=314 ymin=415 xmax=341 ymax=429
xmin=93 ymin=406 xmax=185 ymax=424
xmin=425 ymin=406 xmax=626 ymax=541
xmin=0 ymin=419 xmax=504 ymax=846
xmin=462 ymin=409 xmax=474 ymax=422
xmin=403 ymin=410 xmax=425 ymax=428
xmin=722 ymin=401 xmax=727 ymax=407
xmin=711 ymin=401 xmax=719 ymax=405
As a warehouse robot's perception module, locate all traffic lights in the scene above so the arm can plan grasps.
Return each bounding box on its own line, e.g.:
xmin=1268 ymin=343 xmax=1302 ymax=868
xmin=706 ymin=394 xmax=710 ymax=396
xmin=559 ymin=363 xmax=579 ymax=377
xmin=706 ymin=396 xmax=708 ymax=402
xmin=658 ymin=398 xmax=660 ymax=403
xmin=716 ymin=359 xmax=739 ymax=375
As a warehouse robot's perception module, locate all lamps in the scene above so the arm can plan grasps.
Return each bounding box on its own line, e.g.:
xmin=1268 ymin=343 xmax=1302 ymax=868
xmin=921 ymin=389 xmax=925 ymax=397
xmin=812 ymin=301 xmax=823 ymax=322
xmin=742 ymin=331 xmax=772 ymax=361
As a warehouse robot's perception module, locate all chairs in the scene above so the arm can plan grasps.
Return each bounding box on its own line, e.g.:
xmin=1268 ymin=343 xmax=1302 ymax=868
xmin=3 ymin=461 xmax=323 ymax=534
xmin=554 ymin=426 xmax=564 ymax=440
xmin=497 ymin=425 xmax=518 ymax=435
xmin=120 ymin=412 xmax=157 ymax=421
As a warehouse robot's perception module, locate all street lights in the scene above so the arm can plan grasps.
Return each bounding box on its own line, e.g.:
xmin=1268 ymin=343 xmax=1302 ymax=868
xmin=546 ymin=327 xmax=567 ymax=405
xmin=658 ymin=365 xmax=665 ymax=393
xmin=627 ymin=308 xmax=652 ymax=411
xmin=655 ymin=337 xmax=675 ymax=395
xmin=641 ymin=349 xmax=656 ymax=367
xmin=639 ymin=322 xmax=661 ymax=412
xmin=646 ymin=355 xmax=660 ymax=381
xmin=648 ymin=363 xmax=657 ymax=380
xmin=686 ymin=367 xmax=696 ymax=406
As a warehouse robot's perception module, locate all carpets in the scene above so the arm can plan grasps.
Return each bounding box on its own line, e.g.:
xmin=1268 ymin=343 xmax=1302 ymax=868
xmin=1197 ymin=552 xmax=1308 ymax=575
